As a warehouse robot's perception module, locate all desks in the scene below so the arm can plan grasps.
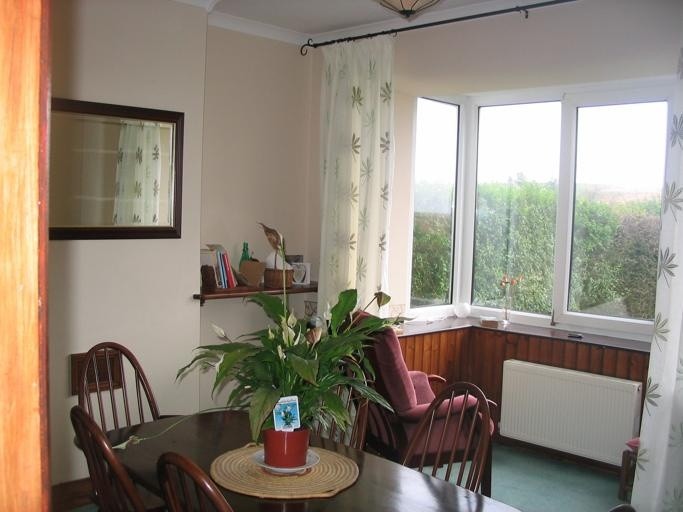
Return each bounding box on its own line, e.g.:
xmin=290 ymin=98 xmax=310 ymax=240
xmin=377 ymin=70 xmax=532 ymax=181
xmin=74 ymin=411 xmax=522 ymax=512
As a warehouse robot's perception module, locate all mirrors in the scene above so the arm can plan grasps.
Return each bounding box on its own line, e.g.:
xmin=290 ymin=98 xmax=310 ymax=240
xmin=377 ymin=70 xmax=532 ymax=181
xmin=50 ymin=97 xmax=185 ymax=241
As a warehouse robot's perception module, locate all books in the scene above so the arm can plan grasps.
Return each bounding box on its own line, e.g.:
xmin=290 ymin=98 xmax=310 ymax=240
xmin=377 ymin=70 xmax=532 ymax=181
xmin=198 ymin=242 xmax=237 ymax=291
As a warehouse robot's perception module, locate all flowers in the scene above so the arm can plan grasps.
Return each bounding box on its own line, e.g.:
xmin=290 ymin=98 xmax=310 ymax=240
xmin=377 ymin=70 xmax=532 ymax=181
xmin=178 ymin=225 xmax=418 ymax=446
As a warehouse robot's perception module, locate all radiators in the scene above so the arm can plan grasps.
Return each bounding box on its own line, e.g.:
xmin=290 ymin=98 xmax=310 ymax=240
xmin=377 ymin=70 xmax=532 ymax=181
xmin=500 ymin=359 xmax=642 ymax=469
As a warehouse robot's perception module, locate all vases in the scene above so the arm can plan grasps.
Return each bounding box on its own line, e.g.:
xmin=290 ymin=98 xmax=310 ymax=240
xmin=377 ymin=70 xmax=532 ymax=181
xmin=262 ymin=430 xmax=311 ymax=466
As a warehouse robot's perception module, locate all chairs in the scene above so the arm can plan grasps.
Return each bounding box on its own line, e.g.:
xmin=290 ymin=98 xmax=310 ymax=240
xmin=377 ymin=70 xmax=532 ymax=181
xmin=400 ymin=382 xmax=492 ymax=500
xmin=300 ymin=356 xmax=368 ymax=448
xmin=347 ymin=311 xmax=497 ymax=500
xmin=80 ymin=341 xmax=182 ymax=500
xmin=69 ymin=404 xmax=174 ymax=512
